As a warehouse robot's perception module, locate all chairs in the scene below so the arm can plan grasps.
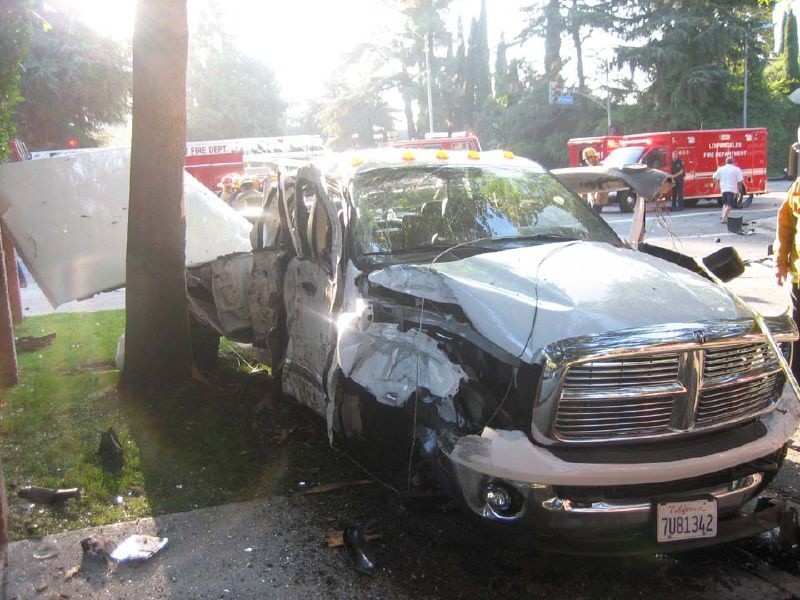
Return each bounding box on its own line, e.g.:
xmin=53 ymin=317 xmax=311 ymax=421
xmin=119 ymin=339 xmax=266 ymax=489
xmin=442 ymin=193 xmax=490 ymax=238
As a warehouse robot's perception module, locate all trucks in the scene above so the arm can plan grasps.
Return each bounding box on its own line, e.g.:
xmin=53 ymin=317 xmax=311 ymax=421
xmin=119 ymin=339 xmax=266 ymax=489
xmin=567 ymin=126 xmax=768 ymax=215
xmin=377 ymin=132 xmax=484 ymax=153
xmin=184 ymin=133 xmax=327 ymax=198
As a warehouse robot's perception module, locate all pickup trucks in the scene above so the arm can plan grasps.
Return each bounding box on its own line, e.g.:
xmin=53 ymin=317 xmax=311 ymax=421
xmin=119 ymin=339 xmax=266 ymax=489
xmin=184 ymin=146 xmax=800 ymax=576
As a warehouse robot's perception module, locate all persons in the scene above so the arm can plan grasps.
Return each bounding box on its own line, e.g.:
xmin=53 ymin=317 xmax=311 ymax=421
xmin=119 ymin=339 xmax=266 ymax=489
xmin=774 ymin=127 xmax=800 ymax=386
xmin=216 ymin=172 xmax=278 ymax=225
xmin=669 ymin=151 xmax=686 ymax=211
xmin=582 ymin=146 xmax=608 ymax=214
xmin=713 ymin=157 xmax=744 ymax=223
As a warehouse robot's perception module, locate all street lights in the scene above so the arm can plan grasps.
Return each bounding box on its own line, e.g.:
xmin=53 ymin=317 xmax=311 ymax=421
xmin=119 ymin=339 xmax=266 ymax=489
xmin=743 ymin=23 xmax=774 ymax=129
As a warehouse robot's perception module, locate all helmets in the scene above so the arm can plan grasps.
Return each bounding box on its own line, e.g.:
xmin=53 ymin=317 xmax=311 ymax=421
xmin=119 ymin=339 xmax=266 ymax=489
xmin=218 ymin=177 xmax=233 ymax=187
xmin=239 ymin=177 xmax=259 ymax=191
xmin=582 ymin=148 xmax=598 ymax=160
